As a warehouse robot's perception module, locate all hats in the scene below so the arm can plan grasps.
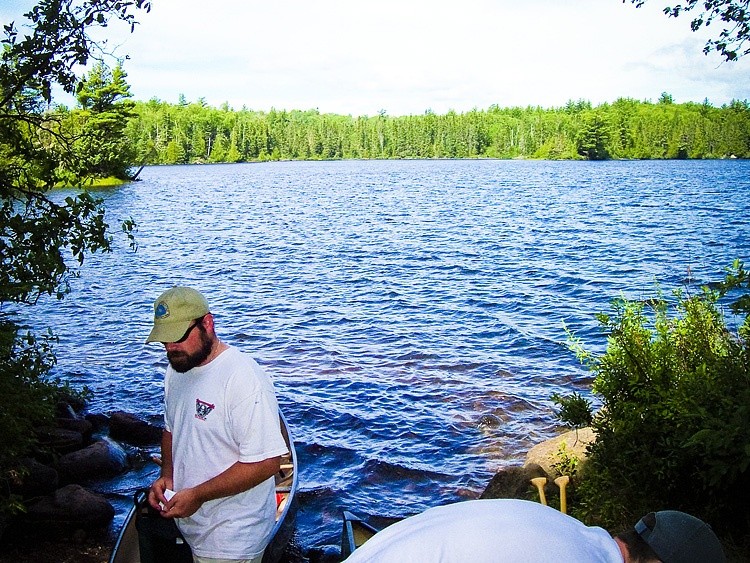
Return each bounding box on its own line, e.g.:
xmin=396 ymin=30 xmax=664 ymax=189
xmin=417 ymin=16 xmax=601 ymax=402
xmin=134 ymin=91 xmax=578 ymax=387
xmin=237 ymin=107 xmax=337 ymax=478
xmin=635 ymin=510 xmax=728 ymax=563
xmin=145 ymin=286 xmax=210 ymax=342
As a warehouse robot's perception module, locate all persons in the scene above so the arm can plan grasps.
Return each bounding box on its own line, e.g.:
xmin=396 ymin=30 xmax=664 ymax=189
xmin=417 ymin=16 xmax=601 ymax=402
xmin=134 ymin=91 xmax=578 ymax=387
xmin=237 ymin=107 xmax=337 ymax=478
xmin=340 ymin=498 xmax=724 ymax=563
xmin=146 ymin=287 xmax=289 ymax=563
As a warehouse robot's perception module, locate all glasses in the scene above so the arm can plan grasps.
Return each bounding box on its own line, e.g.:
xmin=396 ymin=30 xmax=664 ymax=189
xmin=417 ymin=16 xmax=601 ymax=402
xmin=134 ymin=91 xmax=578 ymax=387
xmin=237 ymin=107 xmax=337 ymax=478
xmin=160 ymin=317 xmax=205 ymax=345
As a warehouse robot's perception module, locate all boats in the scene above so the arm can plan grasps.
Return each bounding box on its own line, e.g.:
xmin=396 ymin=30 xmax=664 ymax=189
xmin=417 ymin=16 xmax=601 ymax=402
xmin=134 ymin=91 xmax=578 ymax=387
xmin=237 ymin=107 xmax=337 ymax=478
xmin=109 ymin=403 xmax=298 ymax=563
